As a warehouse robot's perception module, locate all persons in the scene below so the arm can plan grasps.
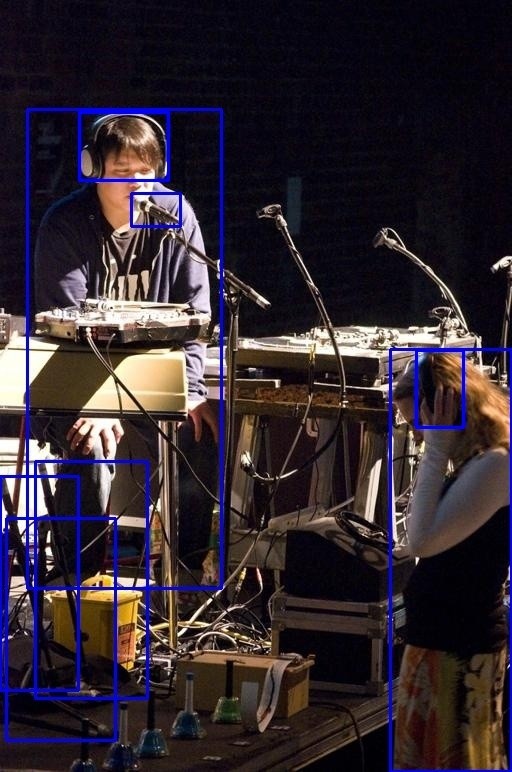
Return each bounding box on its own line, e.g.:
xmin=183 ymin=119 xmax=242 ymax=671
xmin=391 ymin=350 xmax=512 ymax=771
xmin=27 ymin=110 xmax=221 ymax=588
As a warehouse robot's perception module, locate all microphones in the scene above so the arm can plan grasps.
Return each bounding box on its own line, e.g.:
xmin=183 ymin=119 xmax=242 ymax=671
xmin=131 ymin=193 xmax=181 ymax=227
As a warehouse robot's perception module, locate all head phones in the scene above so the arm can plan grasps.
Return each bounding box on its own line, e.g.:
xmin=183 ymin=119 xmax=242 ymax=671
xmin=416 ymin=351 xmax=464 ymax=427
xmin=79 ymin=112 xmax=168 ymax=181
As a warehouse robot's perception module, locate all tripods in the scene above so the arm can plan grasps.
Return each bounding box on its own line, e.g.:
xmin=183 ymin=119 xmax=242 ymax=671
xmin=7 ymin=519 xmax=115 ymax=741
xmin=0 ymin=476 xmax=79 ymax=690
xmin=36 ymin=461 xmax=148 ymax=698
